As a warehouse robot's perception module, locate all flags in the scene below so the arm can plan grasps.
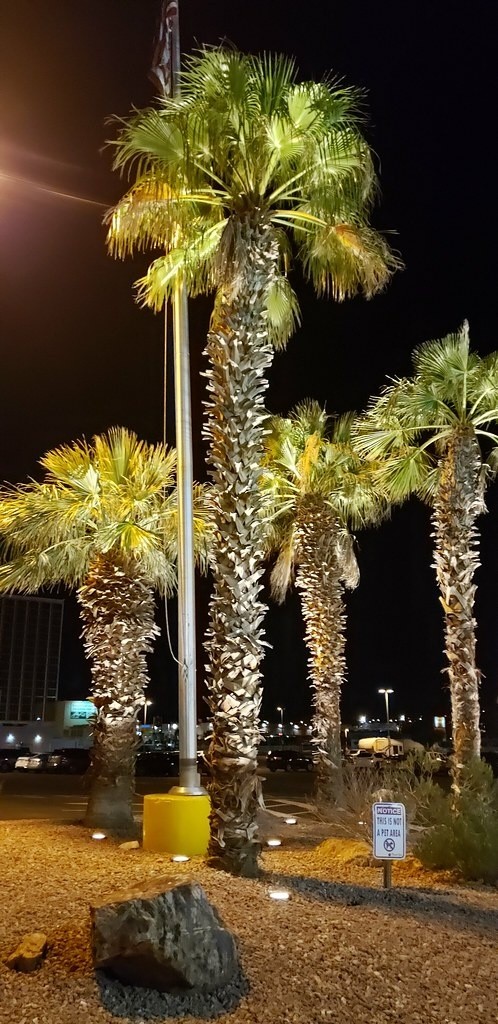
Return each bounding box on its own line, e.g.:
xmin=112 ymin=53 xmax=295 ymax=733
xmin=147 ymin=0 xmax=177 ymax=100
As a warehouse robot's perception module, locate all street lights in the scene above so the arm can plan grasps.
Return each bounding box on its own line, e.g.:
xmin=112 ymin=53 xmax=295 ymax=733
xmin=140 ymin=701 xmax=153 ymax=725
xmin=379 ymin=688 xmax=393 ymax=760
xmin=276 ymin=707 xmax=285 ymax=726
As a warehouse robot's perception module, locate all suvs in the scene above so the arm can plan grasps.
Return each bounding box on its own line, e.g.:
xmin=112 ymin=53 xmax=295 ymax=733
xmin=47 ymin=748 xmax=91 ymax=771
xmin=266 ymin=751 xmax=314 ymax=772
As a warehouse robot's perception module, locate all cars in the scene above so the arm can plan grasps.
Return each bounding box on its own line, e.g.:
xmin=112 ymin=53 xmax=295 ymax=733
xmin=342 ymin=748 xmax=447 ymax=773
xmin=0 ymin=749 xmax=50 ymax=775
xmin=261 ymin=735 xmax=317 ymax=750
xmin=136 ymin=740 xmax=209 ymax=776
